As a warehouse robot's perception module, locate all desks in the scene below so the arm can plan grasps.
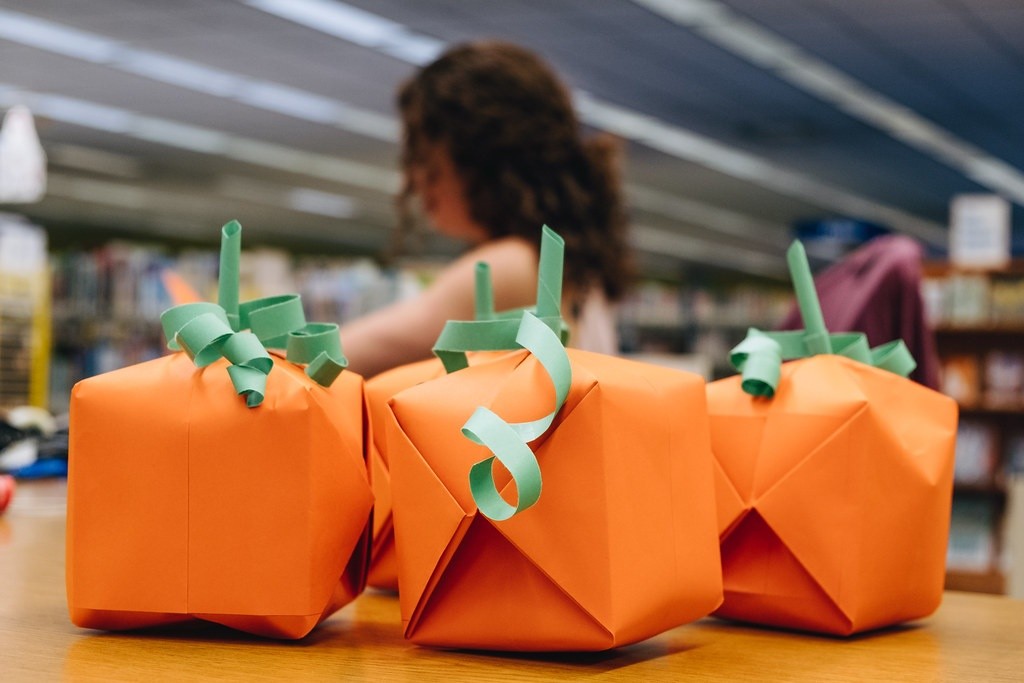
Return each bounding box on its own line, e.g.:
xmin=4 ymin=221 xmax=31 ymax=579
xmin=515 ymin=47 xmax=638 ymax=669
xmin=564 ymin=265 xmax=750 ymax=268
xmin=0 ymin=478 xmax=1024 ymax=683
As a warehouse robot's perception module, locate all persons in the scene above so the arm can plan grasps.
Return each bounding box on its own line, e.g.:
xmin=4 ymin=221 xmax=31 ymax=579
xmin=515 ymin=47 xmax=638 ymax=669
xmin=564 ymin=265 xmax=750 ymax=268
xmin=329 ymin=37 xmax=625 ymax=355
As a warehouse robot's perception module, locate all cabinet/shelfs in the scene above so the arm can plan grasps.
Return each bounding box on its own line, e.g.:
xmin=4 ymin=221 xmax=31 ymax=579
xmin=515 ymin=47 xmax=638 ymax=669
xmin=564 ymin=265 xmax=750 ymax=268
xmin=0 ymin=239 xmax=1023 ymax=592
